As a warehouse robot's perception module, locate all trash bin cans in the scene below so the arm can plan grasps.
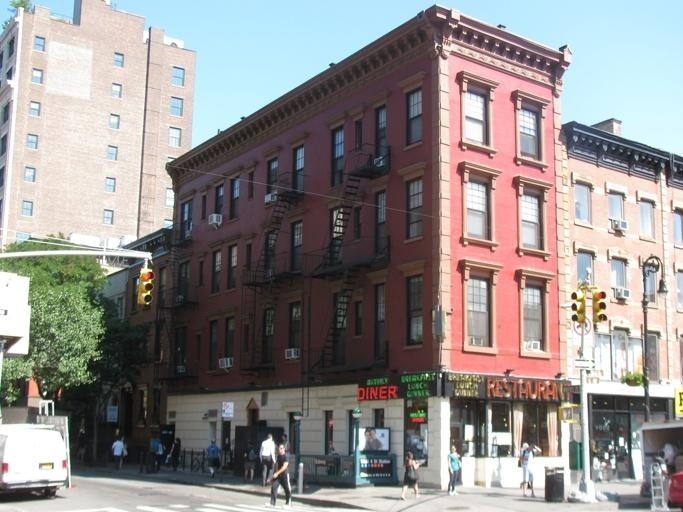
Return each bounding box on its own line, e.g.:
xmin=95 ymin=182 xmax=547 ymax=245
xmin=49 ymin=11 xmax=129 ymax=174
xmin=544 ymin=466 xmax=563 ymax=502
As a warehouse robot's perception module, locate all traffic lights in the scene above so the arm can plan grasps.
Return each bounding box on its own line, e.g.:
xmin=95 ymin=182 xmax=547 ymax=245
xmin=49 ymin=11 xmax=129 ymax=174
xmin=138 ymin=268 xmax=155 ymax=305
xmin=571 ymin=287 xmax=586 ymax=324
xmin=558 ymin=402 xmax=580 ymax=424
xmin=592 ymin=288 xmax=608 ymax=323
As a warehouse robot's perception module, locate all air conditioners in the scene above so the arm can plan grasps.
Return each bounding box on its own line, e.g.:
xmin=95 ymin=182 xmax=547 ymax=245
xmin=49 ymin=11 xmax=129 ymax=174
xmin=375 ymin=156 xmax=384 ymax=168
xmin=527 ymin=340 xmax=541 ymax=352
xmin=208 ymin=213 xmax=223 ymax=226
xmin=284 ymin=348 xmax=300 ymax=359
xmin=218 ymin=357 xmax=233 ymax=369
xmin=265 ymin=192 xmax=277 ymax=205
xmin=614 ymin=287 xmax=630 ymax=301
xmin=176 ymin=365 xmax=185 ymax=373
xmin=611 ymin=217 xmax=626 ymax=230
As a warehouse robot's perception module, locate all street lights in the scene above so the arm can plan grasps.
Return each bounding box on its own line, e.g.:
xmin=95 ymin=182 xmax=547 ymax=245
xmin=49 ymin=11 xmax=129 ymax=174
xmin=352 ymin=407 xmax=362 ymax=487
xmin=640 ymin=255 xmax=668 ymax=422
xmin=293 ymin=411 xmax=303 ymax=483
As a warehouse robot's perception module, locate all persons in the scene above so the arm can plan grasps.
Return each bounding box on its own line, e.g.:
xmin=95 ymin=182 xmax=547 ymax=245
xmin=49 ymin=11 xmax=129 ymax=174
xmin=207 ymin=439 xmax=220 ymax=479
xmin=448 ymin=445 xmax=462 ymax=496
xmin=169 ymin=438 xmax=181 ymax=471
xmin=364 ymin=428 xmax=383 ymax=450
xmin=327 ymin=448 xmax=340 ymax=476
xmin=243 ymin=432 xmax=293 ymax=509
xmin=401 ymin=451 xmax=420 ymax=501
xmin=112 ymin=435 xmax=166 ymax=472
xmin=520 ymin=441 xmax=542 ymax=498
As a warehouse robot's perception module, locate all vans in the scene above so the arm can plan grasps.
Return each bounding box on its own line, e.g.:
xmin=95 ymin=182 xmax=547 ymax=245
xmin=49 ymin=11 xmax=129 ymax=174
xmin=0 ymin=423 xmax=69 ymax=497
